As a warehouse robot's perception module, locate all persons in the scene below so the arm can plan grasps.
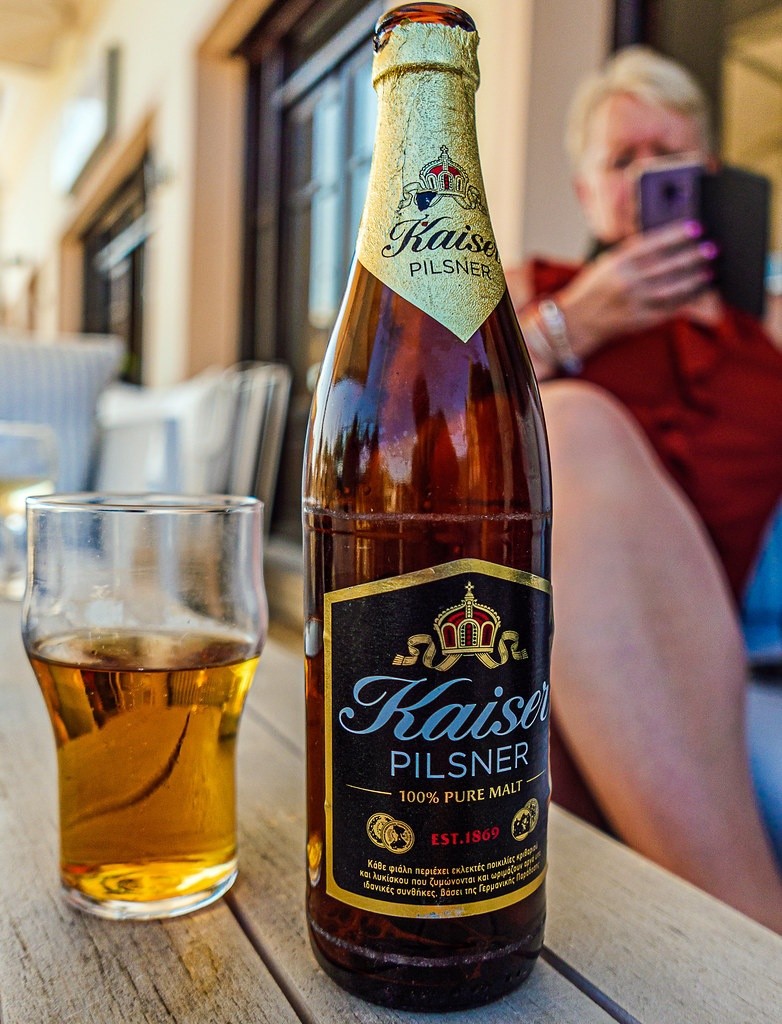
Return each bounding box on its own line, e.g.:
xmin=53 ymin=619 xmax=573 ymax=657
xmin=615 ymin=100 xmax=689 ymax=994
xmin=506 ymin=46 xmax=782 ymax=932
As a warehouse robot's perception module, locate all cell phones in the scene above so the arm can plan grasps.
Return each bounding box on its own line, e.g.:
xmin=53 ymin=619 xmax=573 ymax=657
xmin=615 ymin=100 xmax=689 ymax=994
xmin=633 ymin=155 xmax=705 ymax=268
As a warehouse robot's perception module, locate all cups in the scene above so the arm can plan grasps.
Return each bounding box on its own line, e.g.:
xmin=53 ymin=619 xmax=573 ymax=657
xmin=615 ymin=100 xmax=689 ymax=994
xmin=20 ymin=492 xmax=268 ymax=922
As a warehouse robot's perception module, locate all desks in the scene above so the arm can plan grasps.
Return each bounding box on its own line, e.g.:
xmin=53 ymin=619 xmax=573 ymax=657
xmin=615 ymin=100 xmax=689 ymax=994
xmin=0 ymin=602 xmax=782 ymax=1024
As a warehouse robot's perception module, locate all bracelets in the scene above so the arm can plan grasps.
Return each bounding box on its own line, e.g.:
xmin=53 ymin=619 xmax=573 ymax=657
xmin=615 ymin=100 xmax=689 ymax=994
xmin=526 ymin=302 xmax=582 ymax=376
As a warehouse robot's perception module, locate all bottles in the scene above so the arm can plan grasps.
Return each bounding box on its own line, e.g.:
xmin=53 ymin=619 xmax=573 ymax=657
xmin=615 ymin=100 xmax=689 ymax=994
xmin=298 ymin=2 xmax=557 ymax=1012
